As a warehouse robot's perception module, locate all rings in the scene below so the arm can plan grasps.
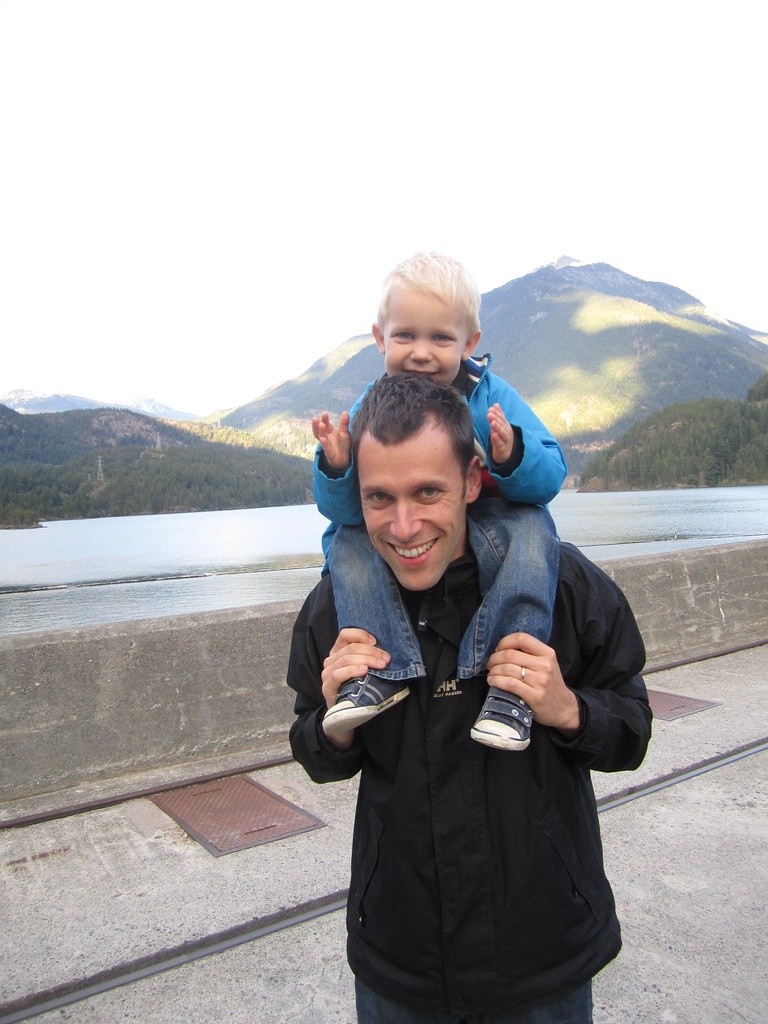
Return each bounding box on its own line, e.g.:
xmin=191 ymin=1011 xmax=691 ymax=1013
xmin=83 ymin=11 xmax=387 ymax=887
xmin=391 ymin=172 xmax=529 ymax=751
xmin=520 ymin=666 xmax=528 ymax=680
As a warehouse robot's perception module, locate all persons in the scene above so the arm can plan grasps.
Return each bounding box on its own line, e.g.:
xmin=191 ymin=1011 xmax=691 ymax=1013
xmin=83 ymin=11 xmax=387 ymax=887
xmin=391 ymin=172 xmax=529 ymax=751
xmin=311 ymin=253 xmax=566 ymax=751
xmin=285 ymin=371 xmax=652 ymax=1024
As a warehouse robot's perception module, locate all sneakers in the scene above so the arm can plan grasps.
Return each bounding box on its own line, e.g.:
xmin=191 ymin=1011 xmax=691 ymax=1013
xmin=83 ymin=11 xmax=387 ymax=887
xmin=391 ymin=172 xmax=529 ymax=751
xmin=470 ymin=686 xmax=535 ymax=751
xmin=322 ymin=673 xmax=410 ymax=735
